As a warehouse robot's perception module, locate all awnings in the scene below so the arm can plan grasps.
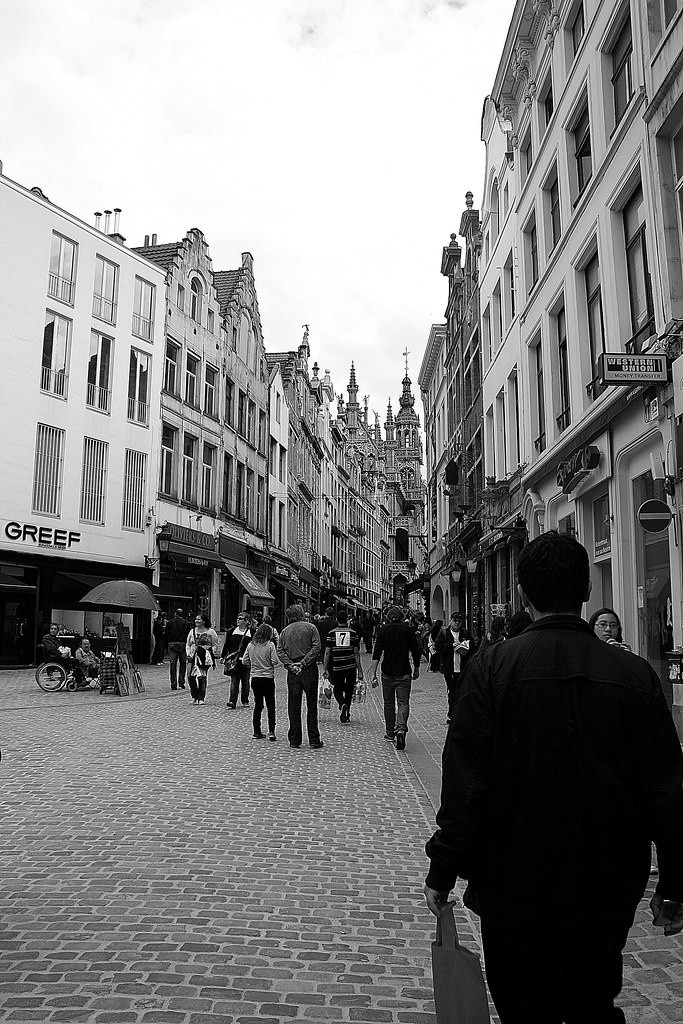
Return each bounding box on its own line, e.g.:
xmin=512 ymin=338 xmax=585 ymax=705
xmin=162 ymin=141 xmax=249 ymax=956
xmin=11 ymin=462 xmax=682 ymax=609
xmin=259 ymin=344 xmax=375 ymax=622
xmin=349 ymin=598 xmax=369 ymax=611
xmin=271 ymin=576 xmax=307 ymax=604
xmin=225 ymin=564 xmax=275 ymax=608
xmin=167 ymin=541 xmax=225 ymax=569
xmin=330 ymin=594 xmax=357 ymax=610
xmin=290 ymin=583 xmax=318 ymax=604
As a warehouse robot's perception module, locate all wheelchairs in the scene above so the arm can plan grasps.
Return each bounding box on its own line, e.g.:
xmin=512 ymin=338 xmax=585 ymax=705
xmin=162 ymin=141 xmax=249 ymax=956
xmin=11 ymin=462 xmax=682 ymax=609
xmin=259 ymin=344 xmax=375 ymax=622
xmin=36 ymin=644 xmax=79 ymax=692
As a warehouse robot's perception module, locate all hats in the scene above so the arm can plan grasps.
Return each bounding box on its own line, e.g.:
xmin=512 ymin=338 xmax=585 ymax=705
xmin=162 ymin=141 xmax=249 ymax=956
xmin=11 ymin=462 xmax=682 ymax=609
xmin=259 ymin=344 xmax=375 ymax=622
xmin=451 ymin=612 xmax=462 ymax=620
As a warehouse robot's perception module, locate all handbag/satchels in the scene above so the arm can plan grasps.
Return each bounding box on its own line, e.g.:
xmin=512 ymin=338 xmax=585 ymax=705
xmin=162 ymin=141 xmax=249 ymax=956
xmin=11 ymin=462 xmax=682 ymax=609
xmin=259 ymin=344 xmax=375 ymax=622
xmin=430 ymin=654 xmax=441 ymax=671
xmin=169 ymin=642 xmax=185 ymax=652
xmin=318 ymin=676 xmax=334 ymax=709
xmin=224 ymin=650 xmax=240 ymax=676
xmin=432 ymin=902 xmax=491 ymax=1024
xmin=192 ymin=647 xmax=213 ymax=670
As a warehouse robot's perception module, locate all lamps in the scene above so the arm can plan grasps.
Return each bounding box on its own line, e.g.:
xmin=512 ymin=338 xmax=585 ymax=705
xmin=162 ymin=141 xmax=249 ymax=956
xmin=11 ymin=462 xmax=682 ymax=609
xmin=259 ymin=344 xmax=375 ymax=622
xmin=451 ymin=559 xmax=463 ymax=582
xmin=452 ymin=504 xmax=479 ymax=523
xmin=486 ymin=514 xmax=526 ymax=531
xmin=407 ymin=534 xmax=426 ymax=576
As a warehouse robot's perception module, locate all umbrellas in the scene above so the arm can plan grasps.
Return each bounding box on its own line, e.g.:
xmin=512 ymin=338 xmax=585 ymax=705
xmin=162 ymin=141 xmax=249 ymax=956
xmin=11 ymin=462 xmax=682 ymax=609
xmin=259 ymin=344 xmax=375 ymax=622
xmin=79 ymin=578 xmax=161 ymax=658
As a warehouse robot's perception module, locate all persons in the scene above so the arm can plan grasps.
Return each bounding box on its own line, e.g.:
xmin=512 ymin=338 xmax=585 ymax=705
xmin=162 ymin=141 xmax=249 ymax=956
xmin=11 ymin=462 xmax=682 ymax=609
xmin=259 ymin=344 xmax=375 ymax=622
xmin=163 ymin=608 xmax=192 ymax=691
xmin=276 ymin=604 xmax=323 ymax=749
xmin=185 ymin=612 xmax=218 ymax=705
xmin=423 ymin=527 xmax=683 ymax=1024
xmin=241 ymin=623 xmax=278 ymax=741
xmin=155 ymin=604 xmax=534 ymax=750
xmin=75 ymin=637 xmax=101 ymax=678
xmin=42 ymin=623 xmax=92 ymax=687
xmin=589 ymin=608 xmax=632 ymax=652
xmin=219 ymin=611 xmax=256 ymax=709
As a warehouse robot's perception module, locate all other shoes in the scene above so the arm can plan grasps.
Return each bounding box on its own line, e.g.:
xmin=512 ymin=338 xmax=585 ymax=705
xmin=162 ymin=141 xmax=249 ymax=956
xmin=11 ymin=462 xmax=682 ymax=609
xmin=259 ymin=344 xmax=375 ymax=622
xmin=340 ymin=703 xmax=350 ymax=723
xmin=157 ymin=663 xmax=164 ymax=665
xmin=227 ymin=703 xmax=235 ymax=709
xmin=397 ymin=731 xmax=406 ymax=750
xmin=179 ymin=685 xmax=185 ymax=688
xmin=269 ymin=732 xmax=276 ymax=740
xmin=290 ymin=745 xmax=299 ymax=748
xmin=384 ymin=735 xmax=394 ymax=742
xmin=252 ymin=733 xmax=266 ymax=738
xmin=241 ymin=703 xmax=249 ymax=707
xmin=190 ymin=698 xmax=205 ymax=705
xmin=310 ymin=742 xmax=323 ymax=747
xmin=172 ymin=687 xmax=177 ymax=690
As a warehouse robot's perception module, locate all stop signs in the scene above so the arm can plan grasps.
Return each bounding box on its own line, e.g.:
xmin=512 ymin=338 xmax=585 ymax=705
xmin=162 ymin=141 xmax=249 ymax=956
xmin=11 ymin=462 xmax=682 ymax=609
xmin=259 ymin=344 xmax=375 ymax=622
xmin=636 ymin=499 xmax=673 ymax=534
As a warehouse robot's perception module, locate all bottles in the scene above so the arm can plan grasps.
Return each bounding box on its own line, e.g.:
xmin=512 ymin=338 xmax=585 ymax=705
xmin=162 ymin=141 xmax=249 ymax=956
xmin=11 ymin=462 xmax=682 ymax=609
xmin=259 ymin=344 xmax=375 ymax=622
xmin=57 ymin=616 xmax=117 ymax=638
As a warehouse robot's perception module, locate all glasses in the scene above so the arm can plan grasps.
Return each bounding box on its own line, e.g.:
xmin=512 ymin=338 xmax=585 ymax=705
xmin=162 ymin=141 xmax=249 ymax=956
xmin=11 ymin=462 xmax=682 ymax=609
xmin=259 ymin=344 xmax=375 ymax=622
xmin=596 ymin=622 xmax=620 ymax=629
xmin=452 ymin=620 xmax=461 ymax=623
xmin=51 ymin=628 xmax=57 ymax=630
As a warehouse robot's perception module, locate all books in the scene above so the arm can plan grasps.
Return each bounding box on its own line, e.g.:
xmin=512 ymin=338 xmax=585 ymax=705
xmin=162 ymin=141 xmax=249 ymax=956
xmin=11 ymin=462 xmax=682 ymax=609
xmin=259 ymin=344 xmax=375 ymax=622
xmin=454 ymin=640 xmax=470 ymax=655
